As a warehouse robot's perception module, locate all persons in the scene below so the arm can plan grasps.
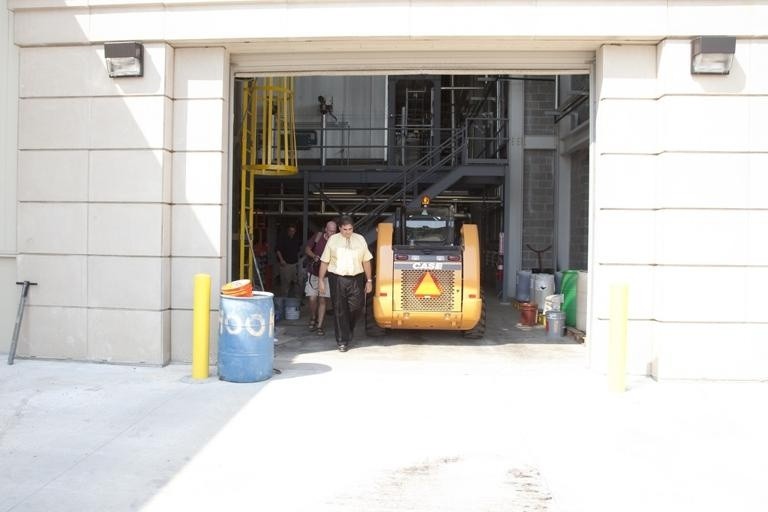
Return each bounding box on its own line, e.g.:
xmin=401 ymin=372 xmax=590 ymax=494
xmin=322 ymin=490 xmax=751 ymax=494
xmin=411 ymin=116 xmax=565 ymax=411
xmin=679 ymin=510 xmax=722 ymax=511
xmin=275 ymin=223 xmax=305 ymax=307
xmin=317 ymin=215 xmax=376 ymax=352
xmin=304 ymin=221 xmax=337 ymax=336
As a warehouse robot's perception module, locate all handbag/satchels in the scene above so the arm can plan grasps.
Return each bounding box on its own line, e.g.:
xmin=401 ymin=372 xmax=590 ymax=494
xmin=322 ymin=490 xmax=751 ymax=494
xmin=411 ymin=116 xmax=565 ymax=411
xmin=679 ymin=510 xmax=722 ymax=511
xmin=302 ymin=231 xmax=323 ymax=267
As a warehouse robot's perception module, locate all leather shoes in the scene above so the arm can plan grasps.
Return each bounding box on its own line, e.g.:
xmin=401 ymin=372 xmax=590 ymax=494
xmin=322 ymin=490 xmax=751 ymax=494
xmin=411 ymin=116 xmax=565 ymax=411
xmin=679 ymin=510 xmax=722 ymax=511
xmin=339 ymin=329 xmax=354 ymax=351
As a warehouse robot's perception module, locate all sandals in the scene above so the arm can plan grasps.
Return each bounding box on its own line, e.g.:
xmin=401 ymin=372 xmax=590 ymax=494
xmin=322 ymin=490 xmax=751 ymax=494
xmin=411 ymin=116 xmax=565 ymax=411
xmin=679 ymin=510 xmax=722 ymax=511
xmin=317 ymin=327 xmax=324 ymax=336
xmin=308 ymin=318 xmax=316 ymax=330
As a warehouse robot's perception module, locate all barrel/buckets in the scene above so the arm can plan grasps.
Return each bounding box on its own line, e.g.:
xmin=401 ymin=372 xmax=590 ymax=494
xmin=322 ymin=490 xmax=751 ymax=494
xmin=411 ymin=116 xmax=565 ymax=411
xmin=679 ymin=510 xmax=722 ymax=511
xmin=283 ymin=298 xmax=301 ymax=320
xmin=219 ymin=291 xmax=275 ymax=382
xmin=221 ymin=279 xmax=253 ymax=297
xmin=517 ymin=268 xmax=589 ymax=337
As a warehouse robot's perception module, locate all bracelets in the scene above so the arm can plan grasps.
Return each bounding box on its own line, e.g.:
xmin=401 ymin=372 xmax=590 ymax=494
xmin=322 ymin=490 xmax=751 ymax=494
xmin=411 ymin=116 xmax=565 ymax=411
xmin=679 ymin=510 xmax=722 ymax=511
xmin=366 ymin=279 xmax=373 ymax=282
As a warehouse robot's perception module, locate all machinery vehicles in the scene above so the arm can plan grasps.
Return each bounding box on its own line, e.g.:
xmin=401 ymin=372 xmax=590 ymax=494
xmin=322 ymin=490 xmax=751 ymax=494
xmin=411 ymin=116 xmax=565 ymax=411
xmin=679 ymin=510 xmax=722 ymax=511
xmin=365 ymin=195 xmax=487 ymax=339
